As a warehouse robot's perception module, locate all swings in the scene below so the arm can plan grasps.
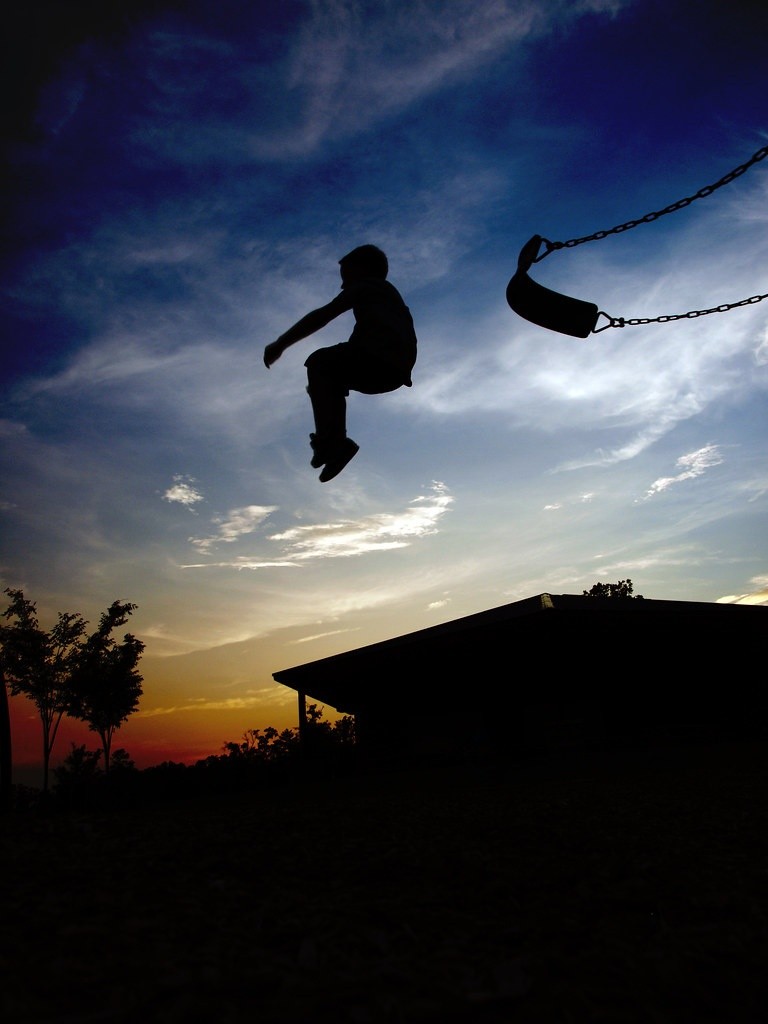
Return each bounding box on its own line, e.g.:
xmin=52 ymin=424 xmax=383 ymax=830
xmin=505 ymin=139 xmax=768 ymax=340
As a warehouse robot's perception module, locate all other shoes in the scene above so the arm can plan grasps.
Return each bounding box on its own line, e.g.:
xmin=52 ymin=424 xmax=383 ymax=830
xmin=309 ymin=433 xmax=343 ymax=468
xmin=319 ymin=437 xmax=360 ymax=483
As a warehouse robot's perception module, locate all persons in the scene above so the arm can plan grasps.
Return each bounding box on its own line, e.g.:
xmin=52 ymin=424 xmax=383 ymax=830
xmin=263 ymin=244 xmax=417 ymax=482
